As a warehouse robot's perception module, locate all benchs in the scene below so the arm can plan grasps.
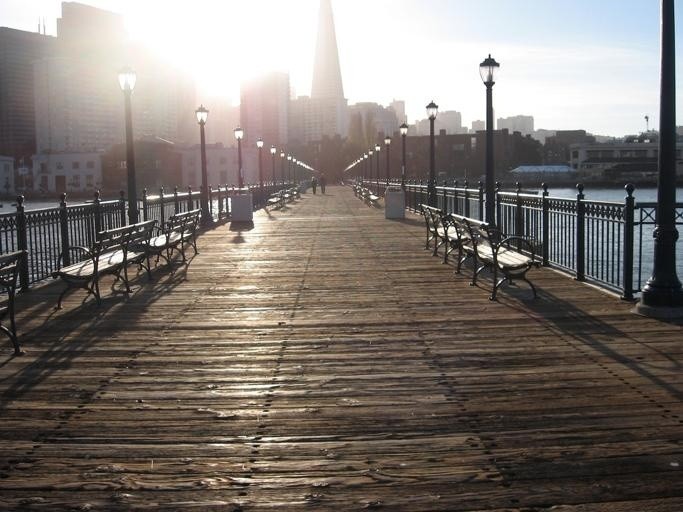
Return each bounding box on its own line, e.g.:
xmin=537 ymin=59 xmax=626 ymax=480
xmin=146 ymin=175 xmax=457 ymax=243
xmin=351 ymin=184 xmax=380 ymax=206
xmin=449 ymin=211 xmax=546 ymax=301
xmin=0 ymin=245 xmax=28 ymax=357
xmin=138 ymin=207 xmax=205 ymax=269
xmin=50 ymin=216 xmax=157 ymax=310
xmin=266 ymin=182 xmax=301 ymax=207
xmin=416 ymin=202 xmax=469 ymax=264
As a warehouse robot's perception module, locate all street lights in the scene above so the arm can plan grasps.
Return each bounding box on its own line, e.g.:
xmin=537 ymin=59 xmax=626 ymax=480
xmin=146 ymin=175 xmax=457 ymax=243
xmin=644 ymin=115 xmax=649 ymax=130
xmin=341 ymin=136 xmax=391 ymax=181
xmin=255 ymin=136 xmax=263 ymax=188
xmin=476 ymin=53 xmax=502 ymax=246
xmin=270 ymin=144 xmax=276 ymax=184
xmin=115 ymin=66 xmax=140 ymax=221
xmin=232 ymin=124 xmax=244 ymax=189
xmin=279 ymin=149 xmax=314 ymax=185
xmin=426 ymin=101 xmax=439 ymax=210
xmin=399 ymin=122 xmax=409 ymax=183
xmin=193 ymin=104 xmax=212 ymax=227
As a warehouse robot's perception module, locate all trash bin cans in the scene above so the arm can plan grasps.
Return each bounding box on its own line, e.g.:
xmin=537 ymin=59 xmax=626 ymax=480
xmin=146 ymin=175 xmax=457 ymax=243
xmin=231 ymin=190 xmax=253 ymax=222
xmin=384 ymin=186 xmax=405 ymax=219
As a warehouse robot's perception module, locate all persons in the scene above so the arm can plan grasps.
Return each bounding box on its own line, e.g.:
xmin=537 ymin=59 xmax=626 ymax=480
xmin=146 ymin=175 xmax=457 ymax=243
xmin=311 ymin=176 xmax=317 ymax=194
xmin=318 ymin=172 xmax=327 ymax=194
